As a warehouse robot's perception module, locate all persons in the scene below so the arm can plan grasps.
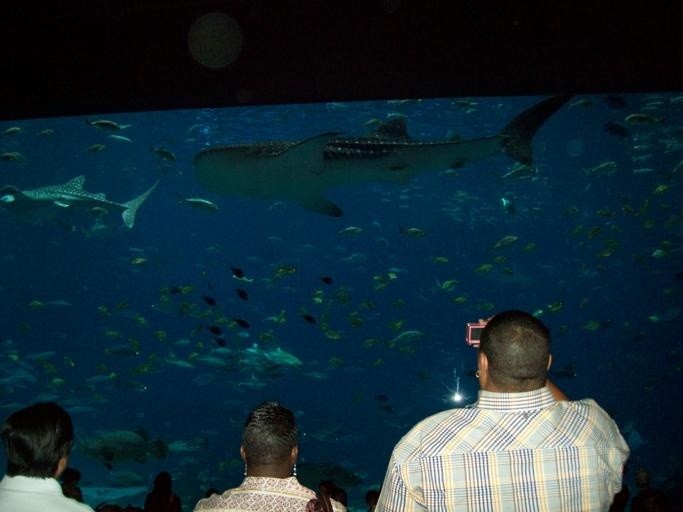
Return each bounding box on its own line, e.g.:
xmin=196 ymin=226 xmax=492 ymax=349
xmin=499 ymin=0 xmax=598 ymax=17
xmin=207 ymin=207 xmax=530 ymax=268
xmin=0 ymin=398 xmax=96 ymax=512
xmin=57 ymin=466 xmax=85 ymax=502
xmin=190 ymin=399 xmax=347 ymax=512
xmin=607 ymin=463 xmax=629 ymax=511
xmin=371 ymin=308 xmax=632 ymax=511
xmin=330 ymin=486 xmax=348 ymax=510
xmin=141 ymin=471 xmax=182 ymax=512
xmin=316 ymin=478 xmax=334 ymax=497
xmin=630 ymin=486 xmax=671 ymax=511
xmin=364 ymin=488 xmax=378 ymax=507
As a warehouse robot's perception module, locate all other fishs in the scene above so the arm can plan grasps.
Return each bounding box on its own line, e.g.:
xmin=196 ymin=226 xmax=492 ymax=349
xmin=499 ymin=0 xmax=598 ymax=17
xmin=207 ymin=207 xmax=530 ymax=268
xmin=0 ymin=94 xmax=682 ymax=512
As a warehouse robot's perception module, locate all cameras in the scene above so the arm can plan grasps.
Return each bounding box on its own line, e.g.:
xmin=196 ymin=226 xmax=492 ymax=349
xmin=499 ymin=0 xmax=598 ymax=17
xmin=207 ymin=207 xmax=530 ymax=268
xmin=466 ymin=323 xmax=486 ymax=346
